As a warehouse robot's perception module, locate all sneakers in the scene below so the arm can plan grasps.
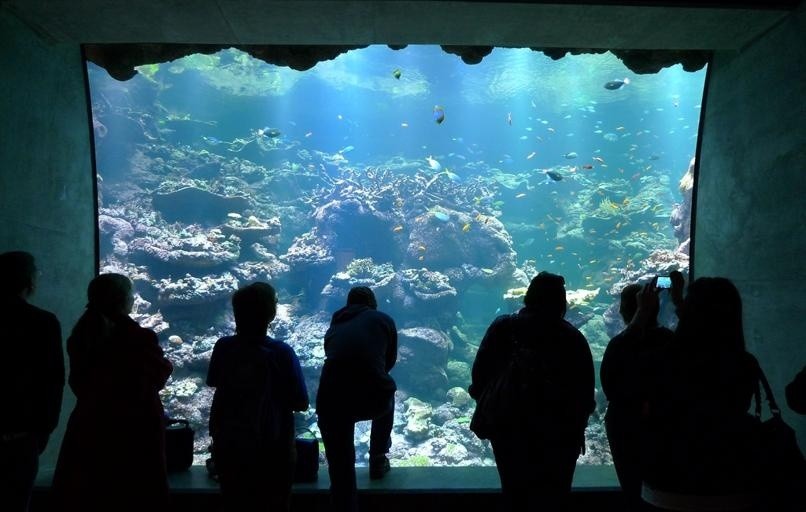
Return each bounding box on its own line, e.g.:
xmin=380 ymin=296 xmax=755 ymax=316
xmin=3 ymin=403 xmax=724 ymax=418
xmin=370 ymin=456 xmax=390 ymax=478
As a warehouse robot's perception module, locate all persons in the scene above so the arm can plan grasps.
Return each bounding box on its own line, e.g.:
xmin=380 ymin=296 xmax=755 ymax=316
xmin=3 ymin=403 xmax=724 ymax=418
xmin=207 ymin=282 xmax=309 ymax=512
xmin=52 ymin=275 xmax=173 ymax=512
xmin=316 ymin=286 xmax=397 ymax=512
xmin=468 ymin=271 xmax=596 ymax=512
xmin=1 ymin=252 xmax=64 ymax=512
xmin=784 ymin=369 xmax=804 ymax=416
xmin=601 ymin=286 xmax=675 ymax=511
xmin=623 ymin=272 xmax=759 ymax=510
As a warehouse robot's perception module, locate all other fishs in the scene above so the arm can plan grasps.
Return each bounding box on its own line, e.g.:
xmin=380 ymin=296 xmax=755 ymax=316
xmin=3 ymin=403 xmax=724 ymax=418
xmin=287 ymin=100 xmax=702 ymax=321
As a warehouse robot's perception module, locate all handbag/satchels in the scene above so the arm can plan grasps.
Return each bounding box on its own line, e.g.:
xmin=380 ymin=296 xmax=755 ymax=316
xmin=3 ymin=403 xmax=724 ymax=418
xmin=762 ymin=416 xmax=803 ymax=461
xmin=165 ymin=419 xmax=194 ymax=472
xmin=295 ymin=428 xmax=319 ymax=481
xmin=468 ymin=373 xmax=508 ymax=440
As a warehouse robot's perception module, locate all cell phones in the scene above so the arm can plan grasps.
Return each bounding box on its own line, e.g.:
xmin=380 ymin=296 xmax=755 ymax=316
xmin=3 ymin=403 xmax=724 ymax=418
xmin=653 ymin=275 xmax=672 ymax=289
